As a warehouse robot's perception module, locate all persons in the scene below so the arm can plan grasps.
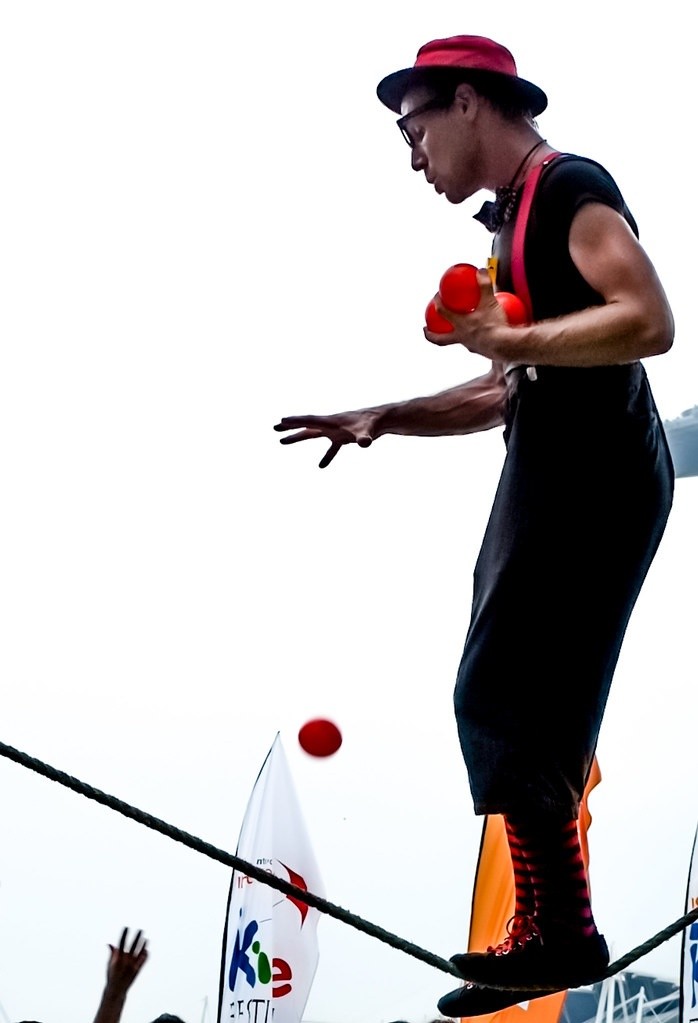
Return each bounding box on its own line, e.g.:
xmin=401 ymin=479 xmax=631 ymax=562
xmin=274 ymin=36 xmax=675 ymax=1019
xmin=92 ymin=928 xmax=147 ymax=1023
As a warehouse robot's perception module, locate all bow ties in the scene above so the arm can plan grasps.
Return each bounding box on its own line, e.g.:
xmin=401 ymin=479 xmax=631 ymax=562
xmin=473 ymin=140 xmax=545 ymax=232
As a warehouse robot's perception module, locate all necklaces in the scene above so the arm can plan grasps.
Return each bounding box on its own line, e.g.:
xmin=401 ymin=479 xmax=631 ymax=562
xmin=485 ymin=138 xmax=549 ymax=230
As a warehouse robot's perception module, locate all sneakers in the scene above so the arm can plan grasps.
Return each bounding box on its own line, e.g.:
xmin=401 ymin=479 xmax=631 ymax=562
xmin=449 ymin=909 xmax=610 ymax=992
xmin=435 ymin=982 xmax=574 ymax=1017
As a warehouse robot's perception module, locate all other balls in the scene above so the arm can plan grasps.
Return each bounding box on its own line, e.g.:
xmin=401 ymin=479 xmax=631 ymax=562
xmin=297 ymin=718 xmax=342 ymax=758
xmin=424 ymin=262 xmax=528 ymax=335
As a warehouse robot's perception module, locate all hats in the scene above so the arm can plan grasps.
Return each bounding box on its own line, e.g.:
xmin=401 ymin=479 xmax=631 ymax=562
xmin=376 ymin=35 xmax=548 ymax=117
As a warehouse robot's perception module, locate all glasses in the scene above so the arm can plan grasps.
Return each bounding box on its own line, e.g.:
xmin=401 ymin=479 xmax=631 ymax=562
xmin=396 ymin=99 xmax=442 ymax=148
